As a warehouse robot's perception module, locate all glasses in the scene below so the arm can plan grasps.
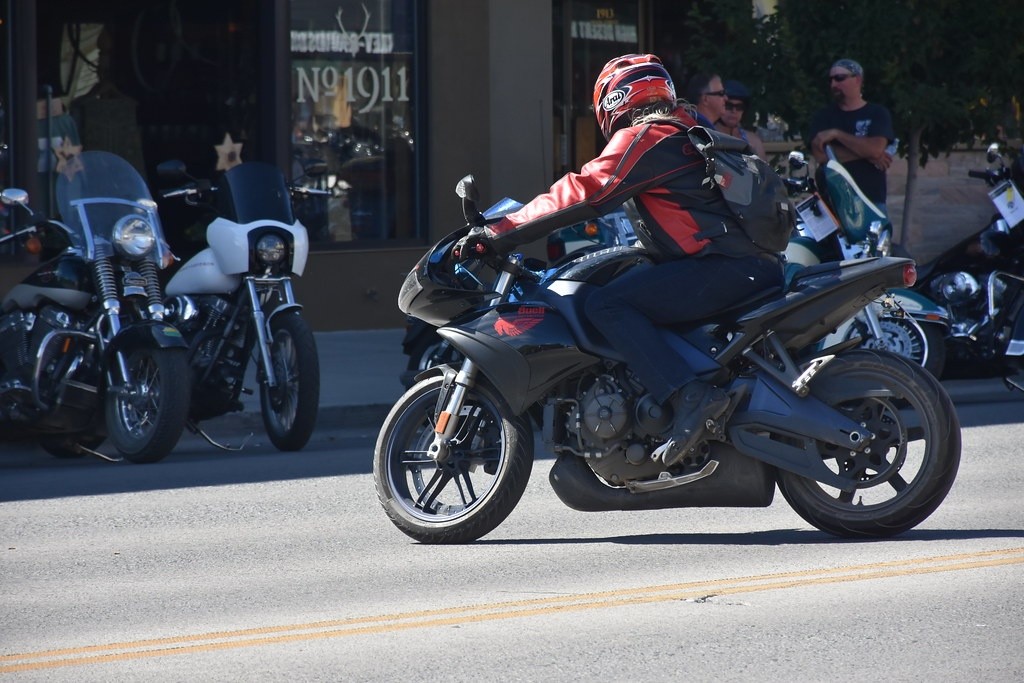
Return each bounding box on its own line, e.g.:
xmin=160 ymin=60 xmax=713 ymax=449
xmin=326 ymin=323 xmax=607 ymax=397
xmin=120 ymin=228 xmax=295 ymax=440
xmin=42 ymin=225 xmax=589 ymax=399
xmin=828 ymin=72 xmax=858 ymax=85
xmin=725 ymin=101 xmax=745 ymax=112
xmin=699 ymin=89 xmax=726 ymax=96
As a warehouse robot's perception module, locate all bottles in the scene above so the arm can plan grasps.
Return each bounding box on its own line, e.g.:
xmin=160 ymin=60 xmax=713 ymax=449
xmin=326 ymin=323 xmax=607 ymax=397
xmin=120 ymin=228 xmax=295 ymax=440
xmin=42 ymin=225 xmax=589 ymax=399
xmin=874 ymin=139 xmax=900 ymax=171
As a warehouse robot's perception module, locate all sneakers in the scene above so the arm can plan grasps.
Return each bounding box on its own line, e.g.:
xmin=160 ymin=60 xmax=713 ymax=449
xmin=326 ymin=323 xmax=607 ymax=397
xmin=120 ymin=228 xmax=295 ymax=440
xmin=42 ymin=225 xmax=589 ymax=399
xmin=661 ymin=380 xmax=730 ymax=467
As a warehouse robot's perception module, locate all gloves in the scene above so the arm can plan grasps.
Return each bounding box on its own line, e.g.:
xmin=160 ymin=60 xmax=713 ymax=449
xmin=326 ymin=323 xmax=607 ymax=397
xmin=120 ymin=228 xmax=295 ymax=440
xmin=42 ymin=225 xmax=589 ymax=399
xmin=451 ymin=227 xmax=498 ymax=263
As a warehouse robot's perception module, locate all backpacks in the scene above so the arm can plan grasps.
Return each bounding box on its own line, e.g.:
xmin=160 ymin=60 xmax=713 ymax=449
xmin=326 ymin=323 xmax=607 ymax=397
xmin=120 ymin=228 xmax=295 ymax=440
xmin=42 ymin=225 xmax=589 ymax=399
xmin=632 ymin=117 xmax=805 ymax=252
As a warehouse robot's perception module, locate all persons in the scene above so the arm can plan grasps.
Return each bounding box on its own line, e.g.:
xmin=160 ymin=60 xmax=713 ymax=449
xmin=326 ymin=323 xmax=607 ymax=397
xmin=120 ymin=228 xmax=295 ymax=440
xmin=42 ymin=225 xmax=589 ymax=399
xmin=681 ymin=70 xmax=767 ymax=165
xmin=449 ymin=52 xmax=786 ymax=466
xmin=800 ymin=59 xmax=894 ymax=222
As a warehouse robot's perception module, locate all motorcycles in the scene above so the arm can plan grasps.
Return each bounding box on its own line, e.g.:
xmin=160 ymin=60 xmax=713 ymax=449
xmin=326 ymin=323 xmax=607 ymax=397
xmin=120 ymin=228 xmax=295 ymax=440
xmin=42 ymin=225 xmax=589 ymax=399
xmin=290 ymin=122 xmax=413 ymax=249
xmin=545 ymin=131 xmax=1024 ymax=408
xmin=0 ymin=147 xmax=336 ymax=468
xmin=370 ymin=172 xmax=963 ymax=546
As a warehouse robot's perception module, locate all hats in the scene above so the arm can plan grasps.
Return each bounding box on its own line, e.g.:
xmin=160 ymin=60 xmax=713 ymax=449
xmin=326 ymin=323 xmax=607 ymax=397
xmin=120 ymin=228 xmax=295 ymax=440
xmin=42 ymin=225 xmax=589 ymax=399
xmin=723 ymin=80 xmax=749 ymax=97
xmin=831 ymin=59 xmax=864 ymax=78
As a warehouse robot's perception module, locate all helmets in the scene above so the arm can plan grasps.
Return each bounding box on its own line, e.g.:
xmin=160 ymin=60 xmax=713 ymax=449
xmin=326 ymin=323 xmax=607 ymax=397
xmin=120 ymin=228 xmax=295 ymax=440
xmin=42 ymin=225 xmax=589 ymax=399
xmin=593 ymin=54 xmax=677 ymax=142
